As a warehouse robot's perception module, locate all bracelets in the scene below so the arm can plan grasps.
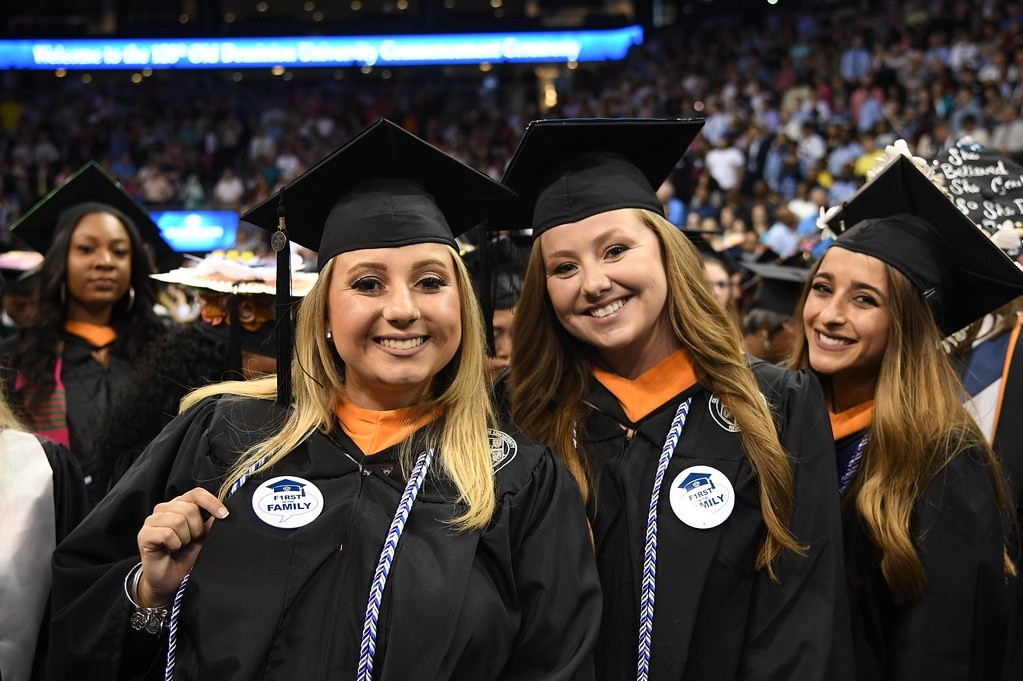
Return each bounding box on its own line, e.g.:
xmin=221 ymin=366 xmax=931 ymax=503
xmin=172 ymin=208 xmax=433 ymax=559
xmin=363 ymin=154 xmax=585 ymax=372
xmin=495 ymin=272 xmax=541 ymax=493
xmin=124 ymin=562 xmax=172 ymax=634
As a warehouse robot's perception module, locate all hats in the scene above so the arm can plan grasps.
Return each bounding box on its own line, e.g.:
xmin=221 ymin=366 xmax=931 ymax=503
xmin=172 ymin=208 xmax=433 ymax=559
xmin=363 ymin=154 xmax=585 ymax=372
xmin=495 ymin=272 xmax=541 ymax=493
xmin=475 ymin=116 xmax=706 ymax=359
xmin=460 ymin=234 xmax=536 ymax=311
xmin=239 ymin=118 xmax=523 ymax=469
xmin=826 ymin=152 xmax=1023 ymax=344
xmin=742 ymin=261 xmax=813 ymax=318
xmin=8 ymin=161 xmax=161 ymax=259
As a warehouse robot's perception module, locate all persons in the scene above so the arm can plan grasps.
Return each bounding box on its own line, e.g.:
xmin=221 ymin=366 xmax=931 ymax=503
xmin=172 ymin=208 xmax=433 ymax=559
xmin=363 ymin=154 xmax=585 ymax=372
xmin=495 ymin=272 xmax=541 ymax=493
xmin=0 ymin=1 xmax=1023 ymax=507
xmin=496 ymin=116 xmax=855 ymax=681
xmin=36 ymin=119 xmax=602 ymax=680
xmin=0 ymin=397 xmax=55 ymax=681
xmin=775 ymin=161 xmax=1023 ymax=681
